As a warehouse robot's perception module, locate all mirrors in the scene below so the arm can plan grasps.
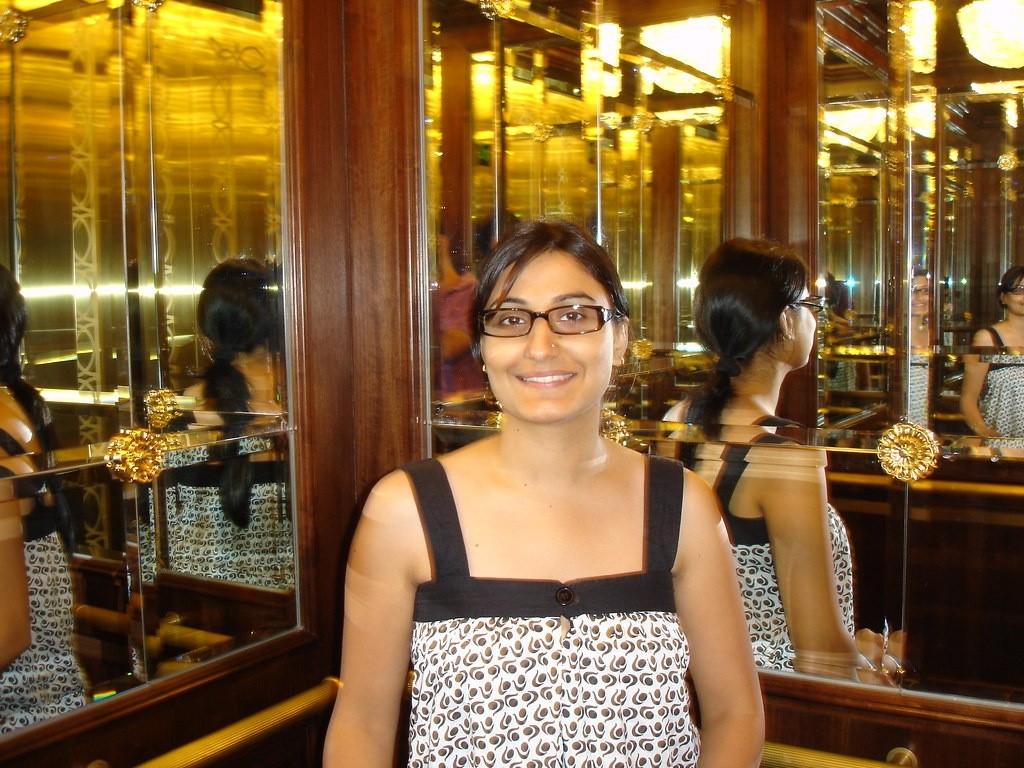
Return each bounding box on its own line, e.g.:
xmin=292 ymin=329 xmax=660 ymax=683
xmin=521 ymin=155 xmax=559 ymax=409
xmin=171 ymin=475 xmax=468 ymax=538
xmin=407 ymin=0 xmax=1024 ymax=732
xmin=0 ymin=0 xmax=317 ymax=768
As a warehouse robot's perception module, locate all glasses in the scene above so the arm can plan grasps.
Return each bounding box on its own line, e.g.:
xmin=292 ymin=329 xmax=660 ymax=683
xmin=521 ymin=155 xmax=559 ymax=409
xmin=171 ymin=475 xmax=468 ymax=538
xmin=1006 ymin=286 xmax=1024 ymax=295
xmin=478 ymin=304 xmax=617 ymax=338
xmin=794 ymin=295 xmax=824 ymax=314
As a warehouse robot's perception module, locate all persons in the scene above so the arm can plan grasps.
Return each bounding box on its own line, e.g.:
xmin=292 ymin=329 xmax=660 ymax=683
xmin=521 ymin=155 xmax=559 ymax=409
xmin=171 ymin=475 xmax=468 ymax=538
xmin=657 ymin=237 xmax=1024 ymax=690
xmin=0 ymin=265 xmax=89 ymax=732
xmin=325 ymin=220 xmax=764 ymax=768
xmin=139 ymin=258 xmax=298 ymax=593
